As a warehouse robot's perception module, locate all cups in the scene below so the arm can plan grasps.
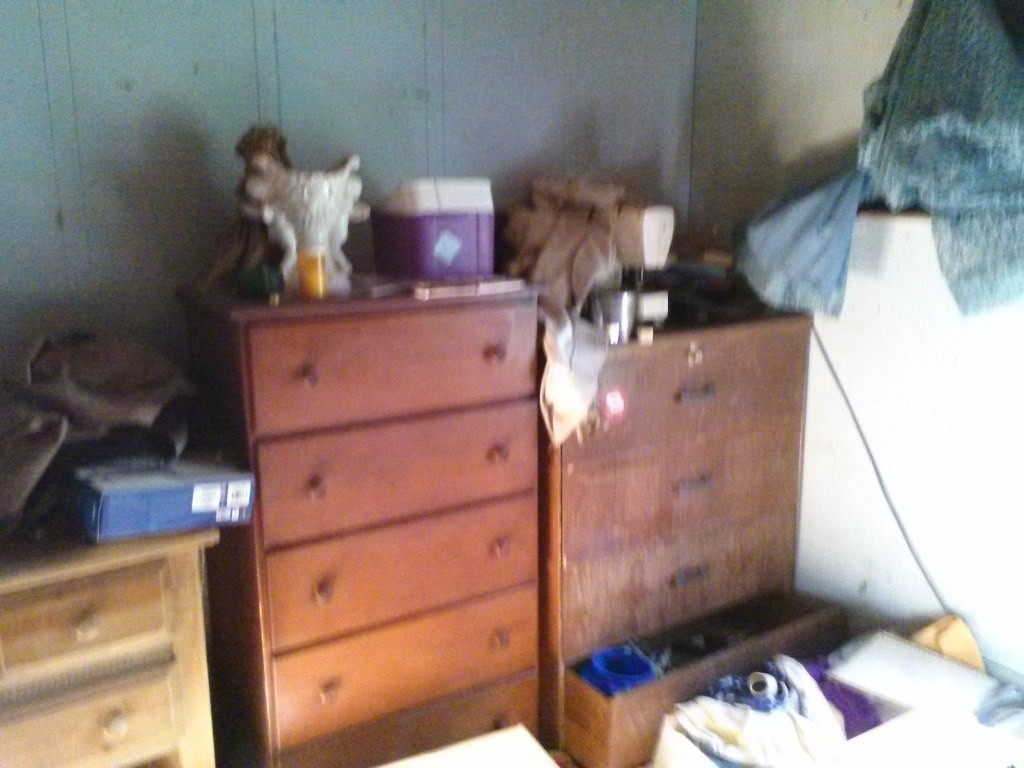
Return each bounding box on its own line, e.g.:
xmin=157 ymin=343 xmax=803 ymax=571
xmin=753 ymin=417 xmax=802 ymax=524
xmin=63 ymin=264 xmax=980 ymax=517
xmin=589 ymin=288 xmax=639 ymax=344
xmin=298 ymin=237 xmax=326 ymax=300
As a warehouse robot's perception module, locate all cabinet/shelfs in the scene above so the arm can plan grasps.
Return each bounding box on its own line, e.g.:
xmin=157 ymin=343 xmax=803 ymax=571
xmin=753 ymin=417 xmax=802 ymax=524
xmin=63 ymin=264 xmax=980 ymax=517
xmin=539 ymin=297 xmax=854 ymax=768
xmin=174 ymin=273 xmax=540 ymax=768
xmin=0 ymin=531 xmax=223 ymax=768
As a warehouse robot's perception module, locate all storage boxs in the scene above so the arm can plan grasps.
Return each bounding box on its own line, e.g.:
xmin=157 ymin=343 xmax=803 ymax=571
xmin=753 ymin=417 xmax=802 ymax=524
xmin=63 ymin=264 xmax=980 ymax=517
xmin=69 ymin=451 xmax=266 ymax=545
xmin=374 ymin=174 xmax=493 ymax=282
